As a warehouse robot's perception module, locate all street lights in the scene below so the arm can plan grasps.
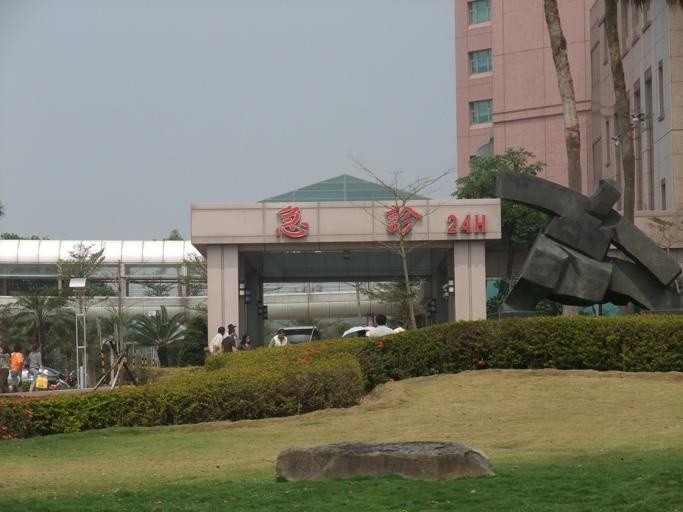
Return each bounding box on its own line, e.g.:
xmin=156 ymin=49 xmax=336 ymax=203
xmin=66 ymin=275 xmax=91 ymax=389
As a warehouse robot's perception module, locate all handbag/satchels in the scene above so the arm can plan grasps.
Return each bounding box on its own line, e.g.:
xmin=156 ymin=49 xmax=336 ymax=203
xmin=35 ymin=374 xmax=48 ymax=389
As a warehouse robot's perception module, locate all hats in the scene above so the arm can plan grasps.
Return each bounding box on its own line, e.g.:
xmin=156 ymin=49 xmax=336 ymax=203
xmin=228 ymin=324 xmax=237 ymax=329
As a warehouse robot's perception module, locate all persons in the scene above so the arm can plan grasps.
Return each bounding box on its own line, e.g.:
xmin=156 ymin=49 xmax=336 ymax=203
xmin=392 ymin=320 xmax=406 ymax=334
xmin=236 ymin=333 xmax=253 ymax=350
xmin=221 ymin=328 xmax=237 ymax=353
xmin=22 ymin=342 xmax=44 ymax=392
xmin=268 ymin=328 xmax=288 ymax=349
xmin=208 ymin=326 xmax=224 ymax=355
xmin=49 ymin=373 xmax=69 ymax=389
xmin=367 ymin=314 xmax=392 ymax=337
xmin=0 ymin=343 xmax=10 ymax=392
xmin=224 ymin=324 xmax=238 ymax=348
xmin=357 ymin=330 xmax=367 ymax=338
xmin=6 ymin=342 xmax=25 ymax=392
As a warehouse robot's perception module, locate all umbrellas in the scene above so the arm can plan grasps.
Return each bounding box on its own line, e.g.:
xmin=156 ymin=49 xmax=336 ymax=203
xmin=340 ymin=325 xmax=375 ymax=338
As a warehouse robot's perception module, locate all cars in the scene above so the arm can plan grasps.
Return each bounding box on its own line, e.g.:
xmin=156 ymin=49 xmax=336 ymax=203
xmin=280 ymin=324 xmax=321 ymax=344
xmin=6 ymin=364 xmax=65 ymax=389
